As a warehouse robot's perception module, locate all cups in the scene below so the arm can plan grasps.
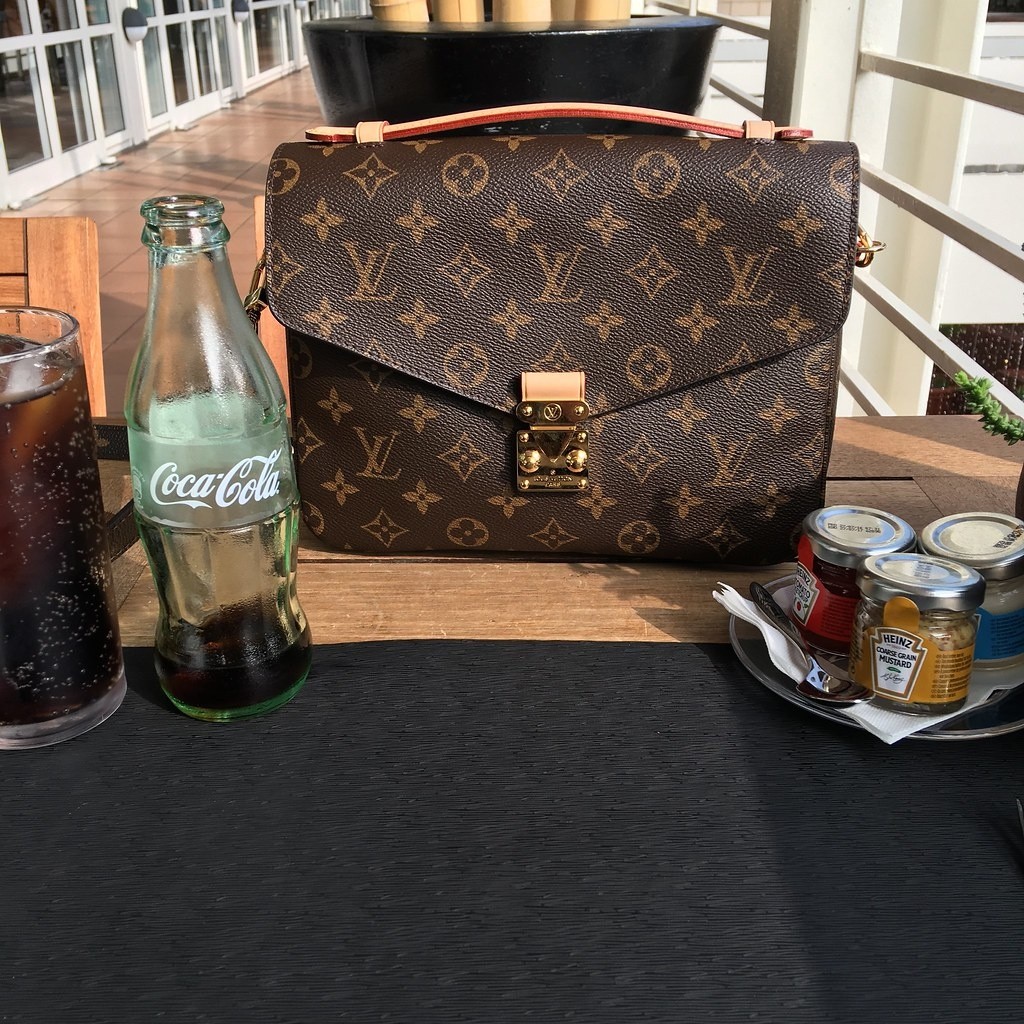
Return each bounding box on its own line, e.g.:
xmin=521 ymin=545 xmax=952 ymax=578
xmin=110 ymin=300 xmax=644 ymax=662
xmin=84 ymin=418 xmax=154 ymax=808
xmin=0 ymin=302 xmax=130 ymax=753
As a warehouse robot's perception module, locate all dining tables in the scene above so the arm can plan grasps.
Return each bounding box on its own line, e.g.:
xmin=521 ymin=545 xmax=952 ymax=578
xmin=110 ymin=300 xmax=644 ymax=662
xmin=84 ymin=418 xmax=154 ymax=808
xmin=0 ymin=390 xmax=1024 ymax=1024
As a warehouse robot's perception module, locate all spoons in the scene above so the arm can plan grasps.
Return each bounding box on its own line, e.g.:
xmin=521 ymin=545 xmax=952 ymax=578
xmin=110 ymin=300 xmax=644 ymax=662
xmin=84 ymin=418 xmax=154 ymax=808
xmin=749 ymin=581 xmax=876 ymax=711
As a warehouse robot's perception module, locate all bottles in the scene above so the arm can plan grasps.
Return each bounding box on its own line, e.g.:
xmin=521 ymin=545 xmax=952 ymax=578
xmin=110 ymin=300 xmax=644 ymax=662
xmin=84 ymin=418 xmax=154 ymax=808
xmin=120 ymin=194 xmax=313 ymax=724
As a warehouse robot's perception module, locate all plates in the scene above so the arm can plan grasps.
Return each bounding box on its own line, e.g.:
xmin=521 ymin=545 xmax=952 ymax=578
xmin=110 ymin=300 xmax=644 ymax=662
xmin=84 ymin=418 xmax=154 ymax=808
xmin=728 ymin=568 xmax=1024 ymax=743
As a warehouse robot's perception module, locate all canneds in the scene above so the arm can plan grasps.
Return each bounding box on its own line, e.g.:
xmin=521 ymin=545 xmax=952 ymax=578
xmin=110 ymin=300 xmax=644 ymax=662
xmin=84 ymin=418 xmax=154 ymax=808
xmin=791 ymin=504 xmax=1024 ymax=711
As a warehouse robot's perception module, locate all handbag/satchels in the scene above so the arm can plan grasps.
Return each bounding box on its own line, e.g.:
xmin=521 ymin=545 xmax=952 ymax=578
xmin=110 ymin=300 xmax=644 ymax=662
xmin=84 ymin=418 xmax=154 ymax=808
xmin=261 ymin=96 xmax=872 ymax=575
